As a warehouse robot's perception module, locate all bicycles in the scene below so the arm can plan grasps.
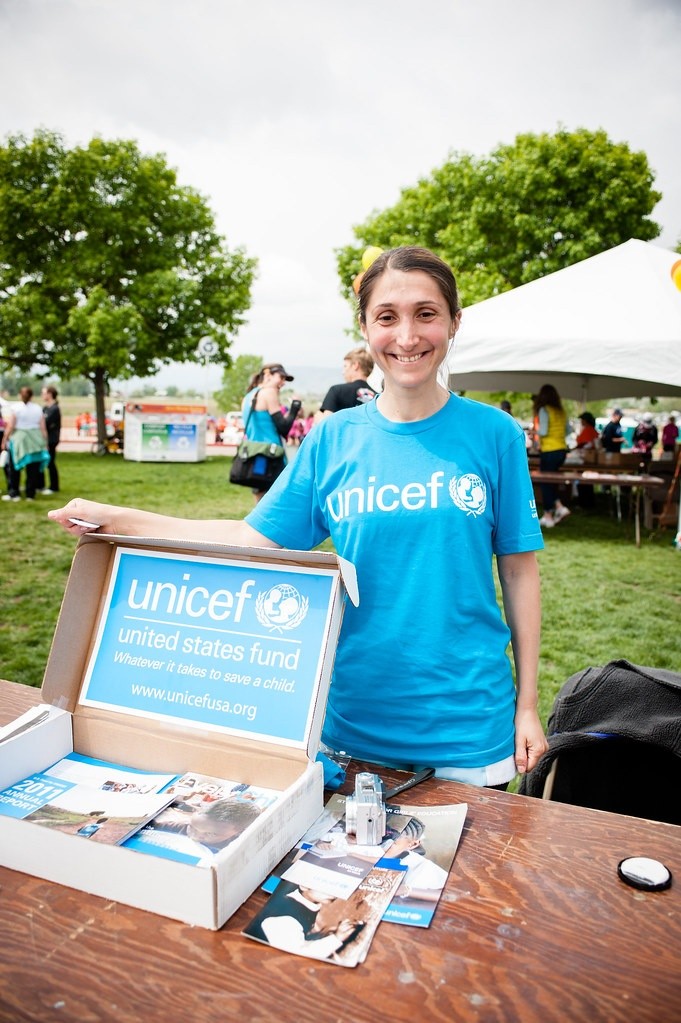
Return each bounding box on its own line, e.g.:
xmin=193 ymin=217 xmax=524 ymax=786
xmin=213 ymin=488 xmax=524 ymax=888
xmin=91 ymin=428 xmax=123 ymax=456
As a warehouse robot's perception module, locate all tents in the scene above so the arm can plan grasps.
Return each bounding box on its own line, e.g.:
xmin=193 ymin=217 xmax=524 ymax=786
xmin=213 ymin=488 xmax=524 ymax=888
xmin=367 ymin=236 xmax=681 ymax=393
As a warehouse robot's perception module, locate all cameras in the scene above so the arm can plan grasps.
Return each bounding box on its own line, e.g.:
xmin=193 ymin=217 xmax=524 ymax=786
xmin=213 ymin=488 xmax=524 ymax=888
xmin=346 ymin=772 xmax=387 ymax=846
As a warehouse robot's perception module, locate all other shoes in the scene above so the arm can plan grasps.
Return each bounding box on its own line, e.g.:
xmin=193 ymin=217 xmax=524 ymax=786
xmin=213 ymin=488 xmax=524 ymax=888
xmin=539 ymin=517 xmax=555 ymax=528
xmin=41 ymin=488 xmax=54 ymax=495
xmin=12 ymin=496 xmax=20 ymax=502
xmin=26 ymin=497 xmax=32 ymax=501
xmin=553 ymin=508 xmax=570 ymax=524
xmin=2 ymin=494 xmax=13 ymax=500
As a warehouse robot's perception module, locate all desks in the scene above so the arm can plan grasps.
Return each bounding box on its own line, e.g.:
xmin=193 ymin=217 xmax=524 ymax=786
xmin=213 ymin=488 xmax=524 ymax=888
xmin=527 ymin=468 xmax=664 ymax=549
xmin=0 ymin=677 xmax=681 ymax=1023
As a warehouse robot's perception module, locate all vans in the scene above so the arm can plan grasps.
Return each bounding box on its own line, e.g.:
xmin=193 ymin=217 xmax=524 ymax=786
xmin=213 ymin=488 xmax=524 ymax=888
xmin=226 ymin=411 xmax=245 ymax=430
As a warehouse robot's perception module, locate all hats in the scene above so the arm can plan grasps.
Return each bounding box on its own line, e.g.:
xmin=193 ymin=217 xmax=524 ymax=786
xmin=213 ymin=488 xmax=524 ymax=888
xmin=262 ymin=364 xmax=294 ymax=381
xmin=615 ymin=410 xmax=623 ymax=417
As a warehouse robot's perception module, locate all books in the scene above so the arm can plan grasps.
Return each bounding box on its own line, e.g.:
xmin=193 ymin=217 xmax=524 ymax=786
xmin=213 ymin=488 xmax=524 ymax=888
xmin=0 ymin=751 xmax=281 ymax=866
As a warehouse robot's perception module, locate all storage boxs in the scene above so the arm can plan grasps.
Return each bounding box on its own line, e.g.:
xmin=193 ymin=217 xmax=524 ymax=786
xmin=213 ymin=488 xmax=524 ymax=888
xmin=0 ymin=535 xmax=361 ymax=933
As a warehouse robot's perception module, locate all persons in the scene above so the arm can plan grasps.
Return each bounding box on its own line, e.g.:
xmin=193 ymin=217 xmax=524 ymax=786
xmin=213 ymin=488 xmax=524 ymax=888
xmin=281 ymin=406 xmax=314 ymax=447
xmin=662 ymin=417 xmax=679 ymax=452
xmin=76 ymin=817 xmax=108 ymax=838
xmin=316 ymin=833 xmax=384 ymax=864
xmin=241 ymin=363 xmax=301 ymax=501
xmin=601 ymin=409 xmax=626 ymax=491
xmin=0 ymin=386 xmax=62 ymax=500
xmin=48 ymin=249 xmax=550 ymax=790
xmin=383 ymin=815 xmax=452 ymax=901
xmin=143 ymin=797 xmax=261 ymax=857
xmin=243 ymin=884 xmax=356 ymax=958
xmin=76 ymin=412 xmax=109 ymax=436
xmin=501 ymin=383 xmax=570 ymax=527
xmin=320 ymin=348 xmax=378 ymax=415
xmin=632 ymin=413 xmax=658 ymax=459
xmin=207 ymin=413 xmax=226 ymax=442
xmin=575 ymin=411 xmax=599 ymax=502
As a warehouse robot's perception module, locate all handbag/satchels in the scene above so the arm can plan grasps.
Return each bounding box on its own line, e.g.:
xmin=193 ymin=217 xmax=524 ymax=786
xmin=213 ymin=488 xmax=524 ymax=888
xmin=229 ymin=441 xmax=286 ymax=489
